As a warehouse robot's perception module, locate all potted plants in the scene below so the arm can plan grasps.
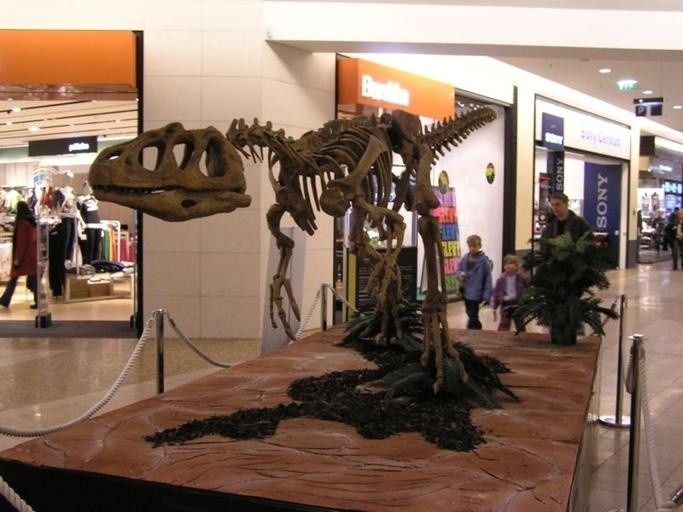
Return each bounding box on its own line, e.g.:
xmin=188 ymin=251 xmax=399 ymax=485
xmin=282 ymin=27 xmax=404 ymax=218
xmin=503 ymin=228 xmax=618 ymax=344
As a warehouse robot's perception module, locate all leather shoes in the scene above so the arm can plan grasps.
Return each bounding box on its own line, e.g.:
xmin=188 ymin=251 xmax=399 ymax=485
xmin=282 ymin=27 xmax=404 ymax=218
xmin=31 ymin=304 xmax=47 ymax=309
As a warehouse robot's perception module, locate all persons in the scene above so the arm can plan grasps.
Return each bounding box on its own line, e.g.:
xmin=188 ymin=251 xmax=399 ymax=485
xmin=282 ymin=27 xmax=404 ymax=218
xmin=456 ymin=235 xmax=491 ymax=329
xmin=638 ymin=204 xmax=682 ymax=273
xmin=491 ymin=256 xmax=532 ymax=332
xmin=537 ymin=192 xmax=597 ymax=335
xmin=0 ymin=201 xmax=39 ymax=310
